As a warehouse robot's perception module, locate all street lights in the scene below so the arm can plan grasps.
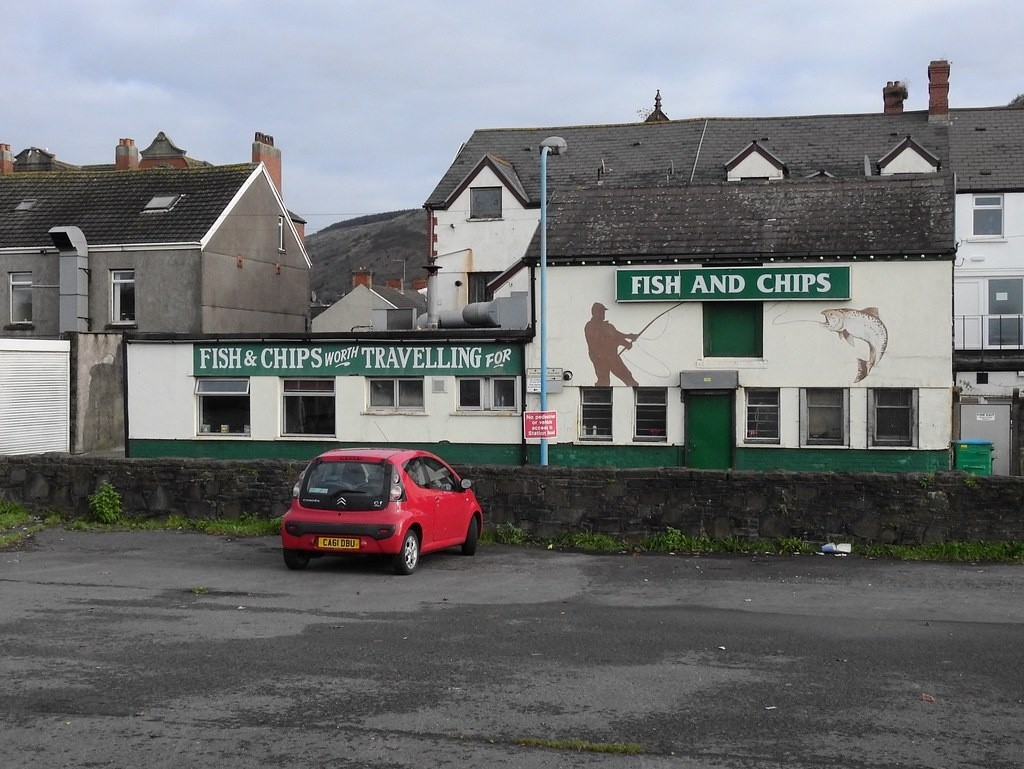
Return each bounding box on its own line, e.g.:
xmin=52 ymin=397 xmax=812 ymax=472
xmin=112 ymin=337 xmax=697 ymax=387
xmin=539 ymin=136 xmax=567 ymax=465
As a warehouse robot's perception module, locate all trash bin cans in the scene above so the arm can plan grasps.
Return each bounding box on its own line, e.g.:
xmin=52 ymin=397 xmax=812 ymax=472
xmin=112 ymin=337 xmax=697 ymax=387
xmin=951 ymin=439 xmax=996 ymax=475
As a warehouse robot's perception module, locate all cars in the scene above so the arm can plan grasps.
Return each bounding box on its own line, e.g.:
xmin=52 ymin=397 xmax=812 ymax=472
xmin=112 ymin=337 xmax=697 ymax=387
xmin=282 ymin=448 xmax=483 ymax=575
